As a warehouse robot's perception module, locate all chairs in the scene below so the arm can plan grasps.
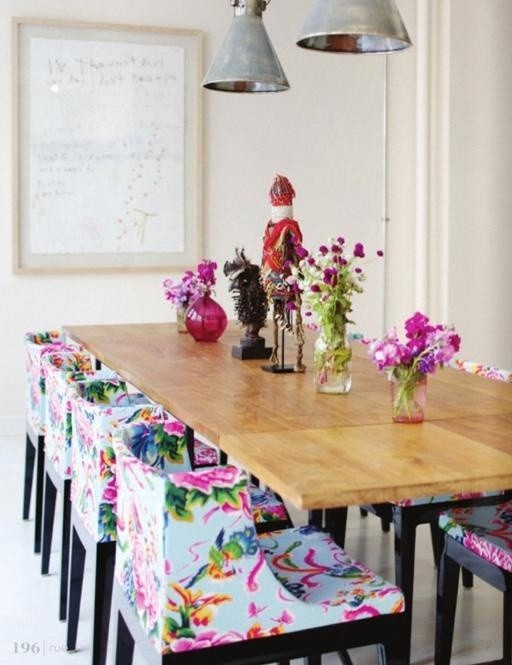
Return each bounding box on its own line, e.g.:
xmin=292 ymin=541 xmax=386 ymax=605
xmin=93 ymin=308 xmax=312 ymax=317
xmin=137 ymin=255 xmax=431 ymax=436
xmin=62 ymin=379 xmax=286 ymax=664
xmin=434 ymin=506 xmax=511 ymax=665
xmin=326 ymin=358 xmax=512 ymax=625
xmin=36 ymin=352 xmax=228 ymax=620
xmin=21 ymin=330 xmax=69 ymax=552
xmin=105 ymin=419 xmax=413 ymax=665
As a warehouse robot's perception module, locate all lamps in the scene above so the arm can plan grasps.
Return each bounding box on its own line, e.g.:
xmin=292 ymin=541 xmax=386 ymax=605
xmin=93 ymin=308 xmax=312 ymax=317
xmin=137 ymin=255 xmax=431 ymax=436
xmin=198 ymin=0 xmax=291 ymax=95
xmin=296 ymin=0 xmax=414 ymax=55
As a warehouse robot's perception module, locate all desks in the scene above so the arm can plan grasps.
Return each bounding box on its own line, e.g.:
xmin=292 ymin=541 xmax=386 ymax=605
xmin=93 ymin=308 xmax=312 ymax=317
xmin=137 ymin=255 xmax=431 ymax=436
xmin=62 ymin=319 xmax=512 ymax=665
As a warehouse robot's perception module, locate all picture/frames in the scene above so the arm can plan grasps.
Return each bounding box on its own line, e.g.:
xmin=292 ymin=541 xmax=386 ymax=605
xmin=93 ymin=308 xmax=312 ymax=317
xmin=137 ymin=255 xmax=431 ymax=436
xmin=9 ymin=14 xmax=206 ymax=276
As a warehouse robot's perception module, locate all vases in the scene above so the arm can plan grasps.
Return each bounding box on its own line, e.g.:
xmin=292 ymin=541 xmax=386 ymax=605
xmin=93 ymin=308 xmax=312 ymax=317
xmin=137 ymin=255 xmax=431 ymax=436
xmin=389 ymin=371 xmax=429 ymax=423
xmin=176 ymin=303 xmax=191 ymax=334
xmin=311 ymin=335 xmax=354 ymax=395
xmin=184 ymin=292 xmax=227 ymax=343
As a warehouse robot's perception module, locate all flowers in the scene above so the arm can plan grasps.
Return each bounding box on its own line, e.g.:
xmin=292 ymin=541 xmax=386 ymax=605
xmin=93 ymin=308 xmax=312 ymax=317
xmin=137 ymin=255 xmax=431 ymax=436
xmin=361 ymin=310 xmax=462 ymax=418
xmin=283 ymin=236 xmax=386 ymax=385
xmin=183 ymin=254 xmax=218 ymax=298
xmin=160 ymin=275 xmax=203 ymax=305
